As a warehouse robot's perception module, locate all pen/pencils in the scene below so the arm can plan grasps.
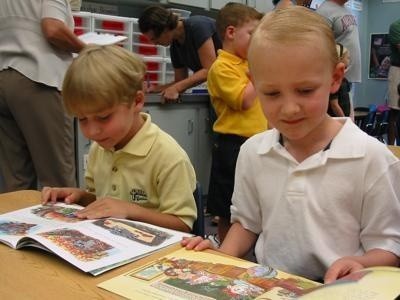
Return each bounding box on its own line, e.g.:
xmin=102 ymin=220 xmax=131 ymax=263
xmin=147 ymin=72 xmax=150 ymax=88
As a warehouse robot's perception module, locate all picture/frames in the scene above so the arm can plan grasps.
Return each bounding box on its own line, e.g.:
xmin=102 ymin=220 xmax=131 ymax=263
xmin=367 ymin=32 xmax=388 ymax=80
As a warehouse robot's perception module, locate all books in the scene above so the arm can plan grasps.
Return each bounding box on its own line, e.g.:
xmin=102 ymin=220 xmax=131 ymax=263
xmin=0 ymin=202 xmax=197 ymax=277
xmin=97 ymin=247 xmax=399 ymax=300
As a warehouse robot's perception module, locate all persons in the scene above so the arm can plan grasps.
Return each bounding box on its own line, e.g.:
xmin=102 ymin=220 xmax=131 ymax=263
xmin=0 ymin=0 xmax=90 ymax=193
xmin=314 ymin=0 xmax=362 ymax=105
xmin=326 ymin=42 xmax=354 ymax=117
xmin=37 ymin=43 xmax=198 ymax=233
xmin=136 ymin=5 xmax=223 ymax=226
xmin=178 ymin=5 xmax=400 ymax=287
xmin=386 ymin=18 xmax=400 ymax=146
xmin=205 ymin=1 xmax=273 ymax=244
xmin=273 ymin=0 xmax=313 ymax=9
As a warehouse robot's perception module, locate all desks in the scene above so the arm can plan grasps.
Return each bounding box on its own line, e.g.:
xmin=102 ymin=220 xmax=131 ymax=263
xmin=0 ymin=189 xmax=243 ymax=300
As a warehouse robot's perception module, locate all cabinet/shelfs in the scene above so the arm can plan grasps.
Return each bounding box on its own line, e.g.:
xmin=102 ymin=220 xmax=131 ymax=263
xmin=149 ymin=108 xmax=213 ymax=196
xmin=72 ymin=9 xmax=176 ymax=87
